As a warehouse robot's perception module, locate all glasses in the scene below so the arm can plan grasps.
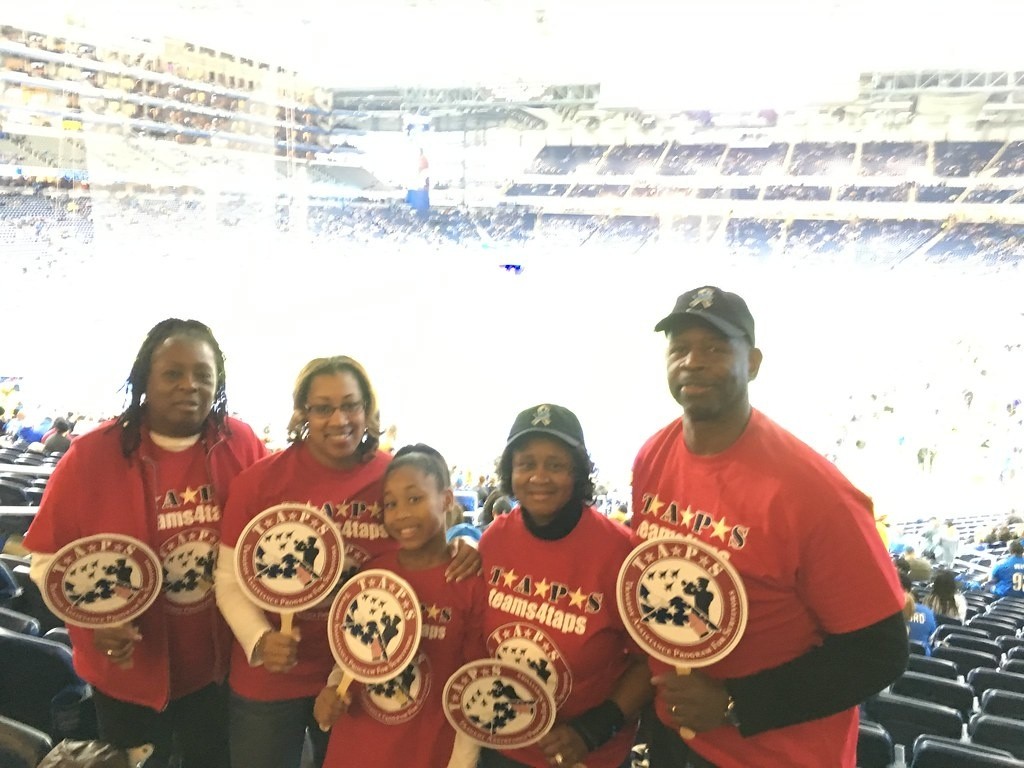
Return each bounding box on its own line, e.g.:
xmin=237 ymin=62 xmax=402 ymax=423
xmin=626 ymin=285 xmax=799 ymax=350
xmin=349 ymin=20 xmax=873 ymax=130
xmin=304 ymin=400 xmax=368 ymax=419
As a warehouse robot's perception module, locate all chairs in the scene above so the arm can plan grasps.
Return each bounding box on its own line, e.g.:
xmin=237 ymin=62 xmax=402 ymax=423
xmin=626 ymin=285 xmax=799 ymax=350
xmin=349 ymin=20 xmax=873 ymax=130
xmin=0 ymin=439 xmax=1024 ymax=768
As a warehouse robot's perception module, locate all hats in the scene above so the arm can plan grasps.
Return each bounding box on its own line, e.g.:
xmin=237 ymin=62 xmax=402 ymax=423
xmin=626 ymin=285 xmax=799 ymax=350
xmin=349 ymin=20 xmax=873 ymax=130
xmin=654 ymin=284 xmax=755 ymax=347
xmin=506 ymin=404 xmax=587 ymax=452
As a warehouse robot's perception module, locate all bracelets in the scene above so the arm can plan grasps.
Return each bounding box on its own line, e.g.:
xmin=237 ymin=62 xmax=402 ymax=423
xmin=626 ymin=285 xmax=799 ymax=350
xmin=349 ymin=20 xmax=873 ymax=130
xmin=568 ymin=699 xmax=626 ymax=753
xmin=722 ymin=695 xmax=735 ymax=720
xmin=253 ymin=633 xmax=264 ymax=659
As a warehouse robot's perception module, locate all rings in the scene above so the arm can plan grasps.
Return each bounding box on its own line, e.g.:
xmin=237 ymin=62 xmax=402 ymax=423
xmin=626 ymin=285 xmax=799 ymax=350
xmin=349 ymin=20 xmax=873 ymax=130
xmin=671 ymin=706 xmax=676 ymax=713
xmin=555 ymin=753 xmax=565 ymax=765
xmin=107 ymin=650 xmax=112 ymax=656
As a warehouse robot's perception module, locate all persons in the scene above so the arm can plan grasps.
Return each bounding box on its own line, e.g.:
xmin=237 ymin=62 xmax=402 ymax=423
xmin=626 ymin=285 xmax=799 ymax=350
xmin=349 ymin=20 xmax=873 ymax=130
xmin=628 ymin=286 xmax=910 ymax=768
xmin=898 ymin=573 xmax=936 ymax=657
xmin=921 ymin=570 xmax=967 ymax=626
xmin=444 ymin=455 xmax=512 ymax=543
xmin=980 ymin=541 xmax=1024 ymax=599
xmin=213 ymin=355 xmax=484 ymax=768
xmin=588 ymin=477 xmax=631 ymax=528
xmin=872 ymin=506 xmax=1024 ymax=591
xmin=311 ymin=442 xmax=486 ymax=768
xmin=21 ymin=317 xmax=266 ymax=768
xmin=0 ymin=401 xmax=119 ymax=454
xmin=475 ymin=402 xmax=653 ymax=768
